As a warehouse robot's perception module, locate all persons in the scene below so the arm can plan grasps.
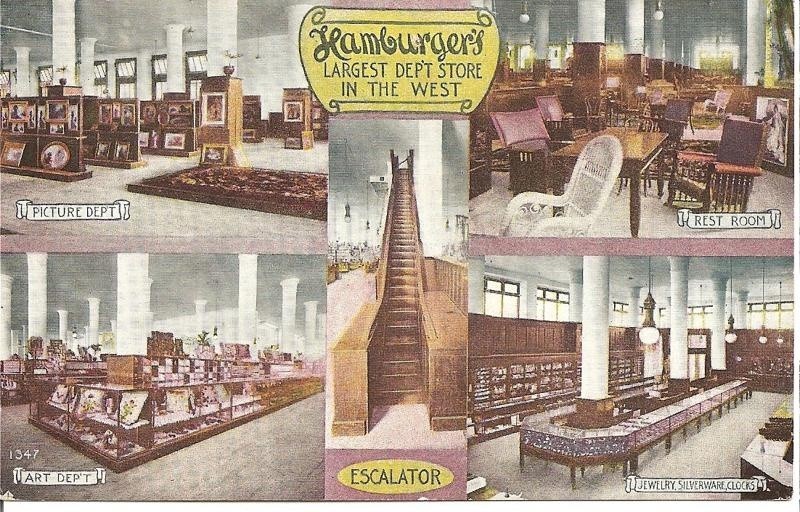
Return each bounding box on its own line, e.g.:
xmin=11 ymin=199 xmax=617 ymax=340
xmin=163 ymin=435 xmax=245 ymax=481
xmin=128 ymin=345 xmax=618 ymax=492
xmin=209 ymin=97 xmax=222 ymax=121
xmin=767 ymin=104 xmax=785 ymax=164
xmin=288 ymin=104 xmax=298 ymax=118
xmin=11 ymin=105 xmax=22 ymax=119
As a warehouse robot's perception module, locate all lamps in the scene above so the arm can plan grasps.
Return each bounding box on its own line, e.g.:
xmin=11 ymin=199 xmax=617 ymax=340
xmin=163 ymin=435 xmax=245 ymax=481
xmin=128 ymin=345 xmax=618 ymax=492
xmin=639 ymin=258 xmax=786 ymax=350
xmin=364 ymin=172 xmax=372 ymax=231
xmin=652 ymin=1 xmax=667 ymax=20
xmin=341 ymin=139 xmax=353 ymax=223
xmin=519 ymin=2 xmax=531 ymax=24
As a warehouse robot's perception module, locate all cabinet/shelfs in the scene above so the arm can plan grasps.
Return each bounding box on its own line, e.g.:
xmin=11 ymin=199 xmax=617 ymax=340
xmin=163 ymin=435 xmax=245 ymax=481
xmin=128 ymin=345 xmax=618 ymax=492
xmin=467 ymin=310 xmax=792 ymax=501
xmin=327 ymin=236 xmax=380 ymax=289
xmin=0 ymin=320 xmax=323 ymax=478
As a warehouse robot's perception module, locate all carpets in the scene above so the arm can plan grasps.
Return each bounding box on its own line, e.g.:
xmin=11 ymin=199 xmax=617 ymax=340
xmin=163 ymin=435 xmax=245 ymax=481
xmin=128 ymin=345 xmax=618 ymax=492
xmin=122 ymin=162 xmax=327 ymax=222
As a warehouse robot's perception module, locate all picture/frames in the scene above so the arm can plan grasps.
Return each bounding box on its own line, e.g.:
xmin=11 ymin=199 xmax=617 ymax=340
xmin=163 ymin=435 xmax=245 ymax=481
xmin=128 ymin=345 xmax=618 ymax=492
xmin=1 ymin=77 xmax=326 ymax=184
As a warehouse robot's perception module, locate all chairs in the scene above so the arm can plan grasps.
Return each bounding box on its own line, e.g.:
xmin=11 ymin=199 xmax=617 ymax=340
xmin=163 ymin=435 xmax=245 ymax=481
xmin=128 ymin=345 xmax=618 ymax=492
xmin=467 ymin=45 xmax=792 ymax=241
